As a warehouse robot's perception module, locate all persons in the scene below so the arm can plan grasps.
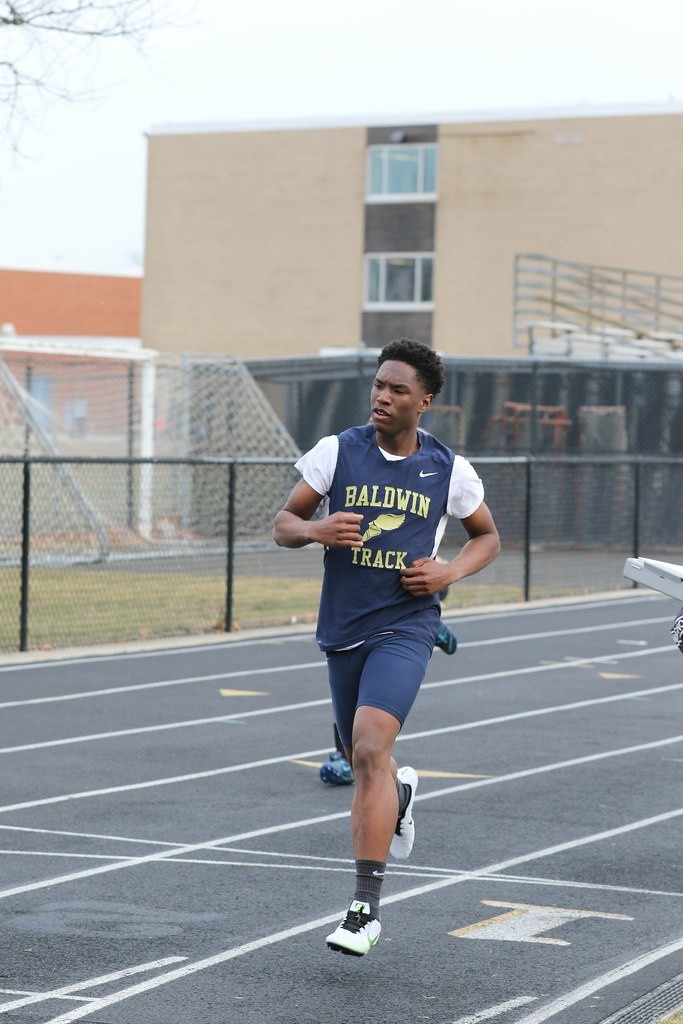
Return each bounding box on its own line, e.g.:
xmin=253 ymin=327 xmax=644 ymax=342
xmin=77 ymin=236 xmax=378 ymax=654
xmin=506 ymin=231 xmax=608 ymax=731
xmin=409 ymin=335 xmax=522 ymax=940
xmin=318 ymin=618 xmax=457 ymax=782
xmin=271 ymin=338 xmax=503 ymax=960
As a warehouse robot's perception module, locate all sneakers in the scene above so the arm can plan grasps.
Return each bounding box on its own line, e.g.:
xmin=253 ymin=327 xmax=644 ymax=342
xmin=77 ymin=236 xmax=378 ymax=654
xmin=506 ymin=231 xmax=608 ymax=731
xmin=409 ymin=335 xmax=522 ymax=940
xmin=320 ymin=751 xmax=355 ymax=785
xmin=388 ymin=766 xmax=418 ymax=859
xmin=433 ymin=621 xmax=457 ymax=655
xmin=324 ymin=899 xmax=381 ymax=957
